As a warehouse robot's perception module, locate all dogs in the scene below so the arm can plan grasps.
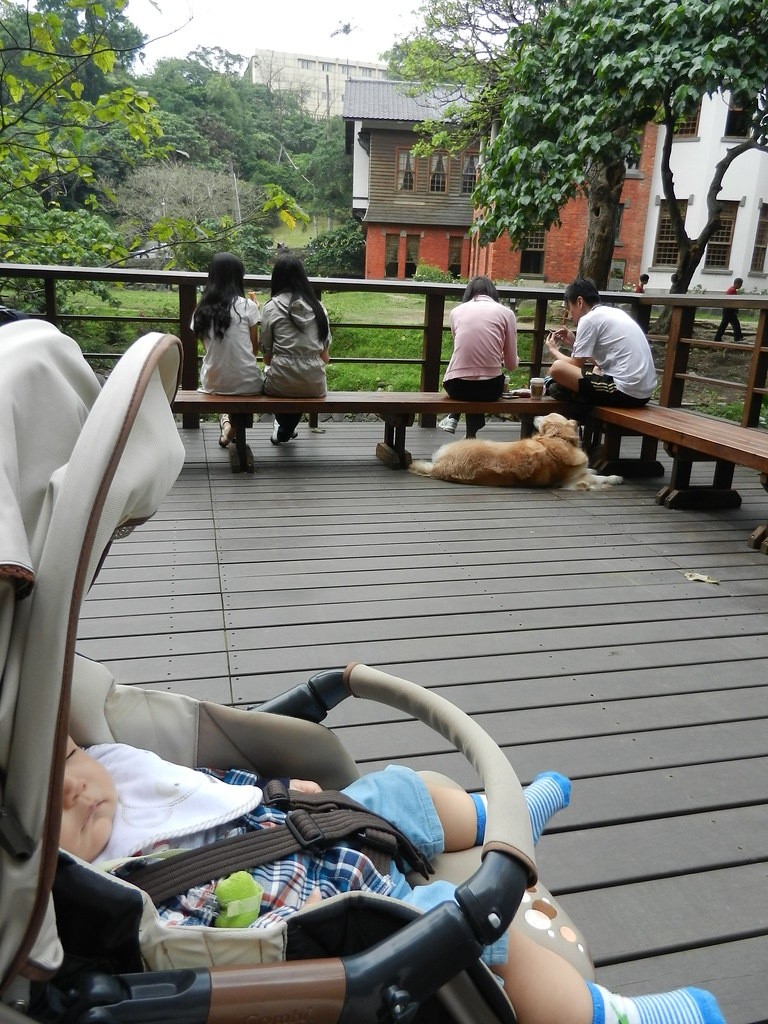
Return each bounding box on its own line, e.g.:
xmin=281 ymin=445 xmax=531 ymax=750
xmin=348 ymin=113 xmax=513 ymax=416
xmin=407 ymin=411 xmax=623 ymax=492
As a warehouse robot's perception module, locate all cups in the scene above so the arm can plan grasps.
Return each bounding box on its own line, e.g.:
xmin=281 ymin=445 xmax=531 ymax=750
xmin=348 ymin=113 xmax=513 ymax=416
xmin=530 ymin=378 xmax=545 ymax=400
xmin=504 ymin=375 xmax=510 ymax=393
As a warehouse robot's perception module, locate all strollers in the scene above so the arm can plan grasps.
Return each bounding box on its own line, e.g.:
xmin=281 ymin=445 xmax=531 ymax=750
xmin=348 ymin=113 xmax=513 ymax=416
xmin=0 ymin=312 xmax=549 ymax=1023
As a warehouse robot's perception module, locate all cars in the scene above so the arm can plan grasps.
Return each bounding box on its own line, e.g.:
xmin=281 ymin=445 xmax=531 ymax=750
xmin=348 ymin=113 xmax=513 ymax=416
xmin=134 ymin=242 xmax=174 ymax=263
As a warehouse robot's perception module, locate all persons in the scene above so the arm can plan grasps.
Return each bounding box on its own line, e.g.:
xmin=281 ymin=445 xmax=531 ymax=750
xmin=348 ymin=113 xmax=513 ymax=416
xmin=541 ymin=277 xmax=658 ymax=408
xmin=259 ymin=252 xmax=333 ymax=447
xmin=438 ymin=276 xmax=520 ymax=442
xmin=635 ymin=274 xmax=650 ymax=295
xmin=188 ymin=252 xmax=264 ymax=447
xmin=713 ymin=278 xmax=746 ymax=342
xmin=58 ymin=731 xmax=725 ymax=1024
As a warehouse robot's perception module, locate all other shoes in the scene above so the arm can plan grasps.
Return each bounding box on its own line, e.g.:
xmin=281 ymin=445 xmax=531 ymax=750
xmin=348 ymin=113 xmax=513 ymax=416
xmin=714 ymin=338 xmax=723 ymax=342
xmin=735 ymin=338 xmax=746 ymax=342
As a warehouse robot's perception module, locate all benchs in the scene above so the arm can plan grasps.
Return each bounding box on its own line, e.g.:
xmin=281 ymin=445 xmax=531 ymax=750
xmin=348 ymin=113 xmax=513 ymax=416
xmin=172 ymin=381 xmax=768 ymax=560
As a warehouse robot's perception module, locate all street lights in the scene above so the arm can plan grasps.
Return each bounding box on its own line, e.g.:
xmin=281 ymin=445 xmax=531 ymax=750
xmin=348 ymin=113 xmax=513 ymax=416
xmin=161 ymin=197 xmax=167 ymax=219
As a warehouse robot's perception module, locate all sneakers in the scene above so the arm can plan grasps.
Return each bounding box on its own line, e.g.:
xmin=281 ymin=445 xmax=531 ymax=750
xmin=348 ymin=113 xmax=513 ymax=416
xmin=270 ymin=418 xmax=281 ymax=445
xmin=545 ymin=375 xmax=590 ymax=404
xmin=219 ymin=413 xmax=232 ymax=447
xmin=439 ymin=413 xmax=458 ymax=434
xmin=288 ymin=423 xmax=299 ymax=439
